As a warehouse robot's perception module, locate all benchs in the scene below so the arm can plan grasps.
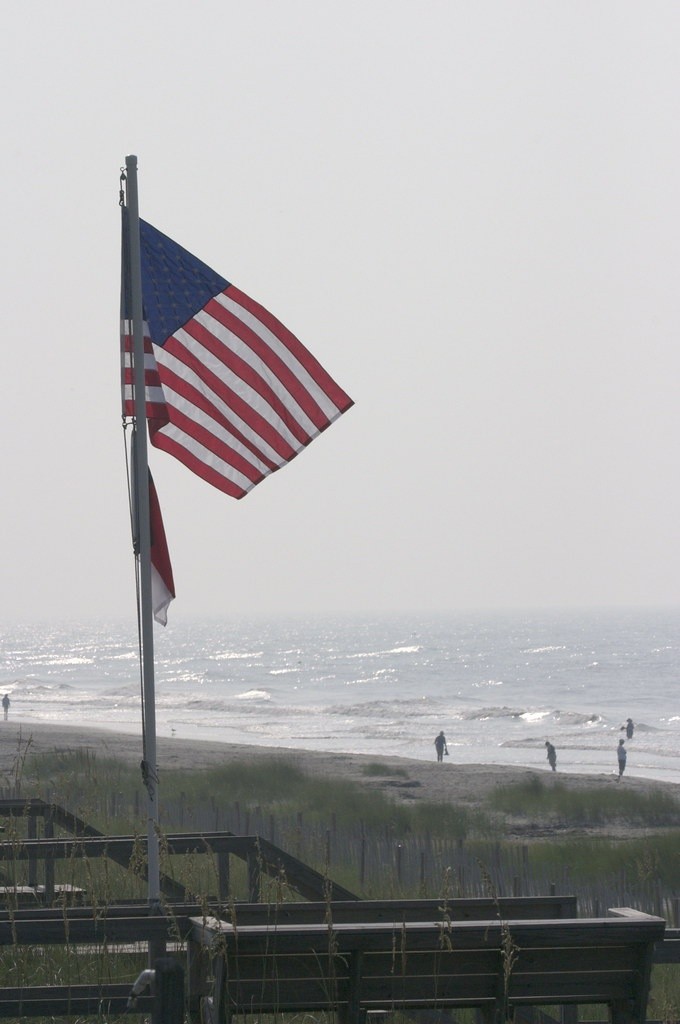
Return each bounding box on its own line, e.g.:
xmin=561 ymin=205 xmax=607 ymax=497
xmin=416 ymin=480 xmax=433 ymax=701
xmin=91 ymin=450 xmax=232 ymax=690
xmin=180 ymin=906 xmax=667 ymax=1024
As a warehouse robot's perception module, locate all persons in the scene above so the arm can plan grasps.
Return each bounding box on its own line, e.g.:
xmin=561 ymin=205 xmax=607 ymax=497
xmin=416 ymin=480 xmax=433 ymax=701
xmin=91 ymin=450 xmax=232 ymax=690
xmin=617 ymin=739 xmax=627 ymax=775
xmin=545 ymin=742 xmax=557 ymax=771
xmin=435 ymin=731 xmax=446 ymax=762
xmin=624 ymin=719 xmax=634 ymax=739
xmin=2 ymin=694 xmax=10 ymax=712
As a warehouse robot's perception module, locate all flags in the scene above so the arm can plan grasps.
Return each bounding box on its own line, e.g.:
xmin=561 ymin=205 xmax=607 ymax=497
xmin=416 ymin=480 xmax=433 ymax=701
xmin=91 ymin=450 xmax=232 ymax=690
xmin=121 ymin=208 xmax=355 ymax=501
xmin=147 ymin=465 xmax=176 ymax=627
